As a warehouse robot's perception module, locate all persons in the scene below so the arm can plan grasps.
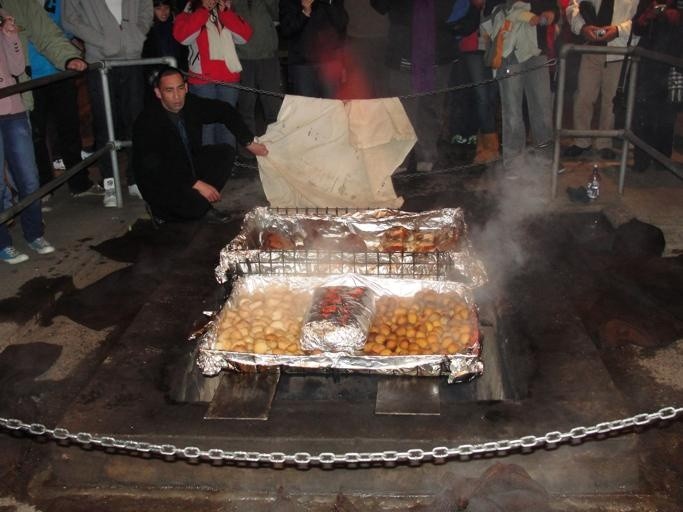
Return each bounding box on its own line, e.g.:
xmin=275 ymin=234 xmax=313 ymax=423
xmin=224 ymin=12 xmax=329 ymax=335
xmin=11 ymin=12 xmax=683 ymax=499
xmin=1 ymin=1 xmax=682 ymax=265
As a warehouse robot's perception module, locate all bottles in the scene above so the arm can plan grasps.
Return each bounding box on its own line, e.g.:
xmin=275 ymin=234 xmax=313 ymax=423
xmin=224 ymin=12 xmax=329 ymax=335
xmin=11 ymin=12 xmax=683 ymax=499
xmin=595 ymin=28 xmax=606 ymax=37
xmin=584 ymin=164 xmax=602 ymax=199
xmin=537 ymin=15 xmax=548 ymax=26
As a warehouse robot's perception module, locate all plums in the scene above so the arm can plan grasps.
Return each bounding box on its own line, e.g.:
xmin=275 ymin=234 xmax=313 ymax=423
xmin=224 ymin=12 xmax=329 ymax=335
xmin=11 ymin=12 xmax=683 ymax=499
xmin=364 ymin=290 xmax=471 ymax=357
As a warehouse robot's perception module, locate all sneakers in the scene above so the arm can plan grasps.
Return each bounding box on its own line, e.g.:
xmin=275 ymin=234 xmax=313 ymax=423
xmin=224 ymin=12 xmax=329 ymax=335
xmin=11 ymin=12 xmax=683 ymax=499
xmin=127 ymin=183 xmax=143 ymax=200
xmin=504 ymin=161 xmax=532 ymax=180
xmin=535 ymin=156 xmax=566 ymax=175
xmin=25 ymin=233 xmax=55 ymax=255
xmin=597 ymin=147 xmax=618 ymax=160
xmin=203 ymin=207 xmax=232 ymax=224
xmin=565 ymin=144 xmax=593 ymax=158
xmin=0 ymin=245 xmax=30 ymax=265
xmin=39 ymin=149 xmax=118 ymax=213
xmin=145 ymin=203 xmax=169 ymax=231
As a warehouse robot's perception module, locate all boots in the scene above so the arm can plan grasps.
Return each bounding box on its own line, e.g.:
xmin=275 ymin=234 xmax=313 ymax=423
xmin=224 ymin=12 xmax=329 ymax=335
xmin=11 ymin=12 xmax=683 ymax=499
xmin=472 ymin=131 xmax=500 ymax=166
